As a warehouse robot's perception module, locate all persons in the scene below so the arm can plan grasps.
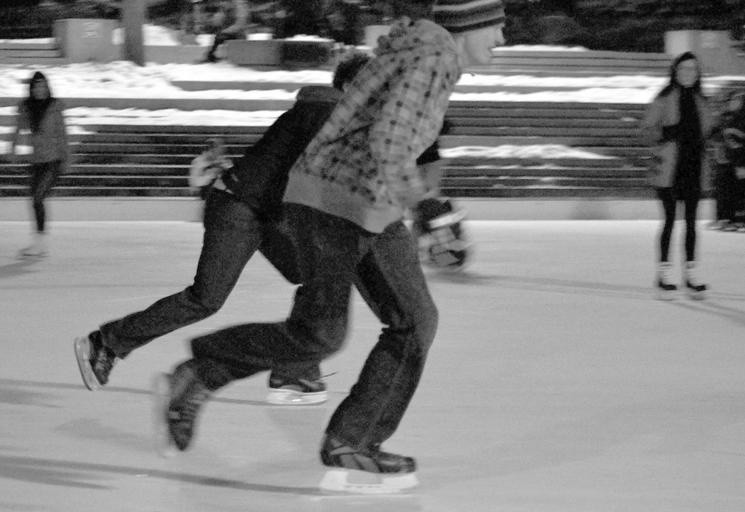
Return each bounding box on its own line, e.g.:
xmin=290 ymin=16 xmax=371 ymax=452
xmin=6 ymin=69 xmax=68 ymax=260
xmin=717 ymin=88 xmax=745 ymax=234
xmin=639 ymin=51 xmax=717 ymax=297
xmin=706 ymin=109 xmax=736 ymax=230
xmin=188 ymin=136 xmax=234 ymax=201
xmin=412 ymin=116 xmax=470 ymax=268
xmin=82 ymin=51 xmax=371 ymax=397
xmin=198 ymin=0 xmax=248 ymax=62
xmin=162 ymin=0 xmax=509 ymax=479
xmin=268 ymin=0 xmax=320 ymax=40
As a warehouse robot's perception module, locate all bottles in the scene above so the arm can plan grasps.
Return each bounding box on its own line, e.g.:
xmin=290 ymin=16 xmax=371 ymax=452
xmin=426 ymin=0 xmax=506 ymax=31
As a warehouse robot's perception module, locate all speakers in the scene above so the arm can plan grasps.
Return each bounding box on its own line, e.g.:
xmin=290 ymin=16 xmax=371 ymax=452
xmin=17 ymin=231 xmax=53 ymax=256
xmin=681 ymin=260 xmax=710 ymax=291
xmin=654 ymin=260 xmax=678 ymax=289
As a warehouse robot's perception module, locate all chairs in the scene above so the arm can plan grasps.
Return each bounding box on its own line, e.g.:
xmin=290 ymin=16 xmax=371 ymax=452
xmin=86 ymin=329 xmax=113 ymax=385
xmin=161 ymin=351 xmax=221 ymax=453
xmin=267 ymin=374 xmax=327 ymax=393
xmin=317 ymin=428 xmax=422 ymax=477
